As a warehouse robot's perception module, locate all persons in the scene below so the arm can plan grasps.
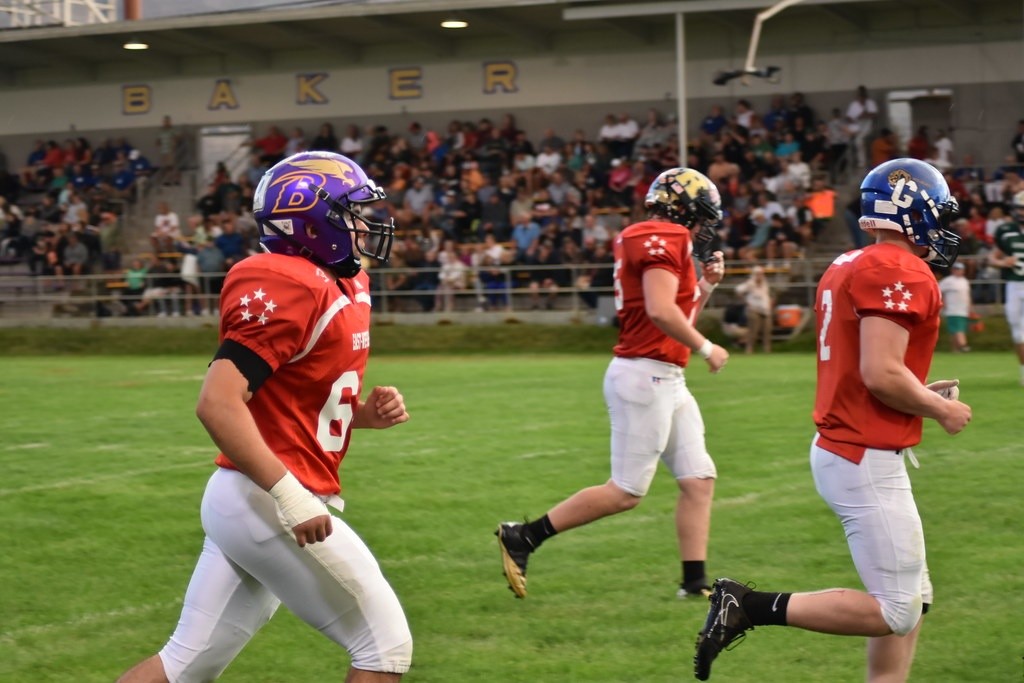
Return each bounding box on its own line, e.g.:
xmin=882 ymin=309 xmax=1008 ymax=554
xmin=0 ymin=83 xmax=1024 ymax=373
xmin=497 ymin=168 xmax=731 ymax=604
xmin=115 ymin=147 xmax=413 ymax=681
xmin=694 ymin=157 xmax=974 ymax=683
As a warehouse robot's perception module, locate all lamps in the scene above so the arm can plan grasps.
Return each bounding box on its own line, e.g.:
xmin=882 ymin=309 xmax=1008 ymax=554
xmin=440 ymin=12 xmax=468 ymax=28
xmin=122 ymin=33 xmax=148 ymax=50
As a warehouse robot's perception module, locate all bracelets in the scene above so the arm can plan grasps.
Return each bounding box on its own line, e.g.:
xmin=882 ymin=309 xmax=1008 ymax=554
xmin=697 ymin=339 xmax=712 ymax=360
xmin=698 ymin=276 xmax=718 ymax=293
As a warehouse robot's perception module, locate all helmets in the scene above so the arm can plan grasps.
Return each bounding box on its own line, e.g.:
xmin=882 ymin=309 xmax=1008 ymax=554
xmin=858 ymin=157 xmax=962 ymax=269
xmin=253 ymin=150 xmax=395 ymax=279
xmin=644 ymin=167 xmax=723 ymax=264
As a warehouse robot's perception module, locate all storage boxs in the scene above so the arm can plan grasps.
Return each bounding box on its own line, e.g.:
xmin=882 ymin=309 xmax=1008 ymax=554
xmin=802 ymin=189 xmax=837 ymax=219
xmin=775 ymin=304 xmax=801 ymax=328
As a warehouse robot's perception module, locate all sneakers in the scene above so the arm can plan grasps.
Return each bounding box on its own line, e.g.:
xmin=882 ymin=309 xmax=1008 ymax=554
xmin=493 ymin=522 xmax=529 ymax=601
xmin=675 ymin=582 xmax=713 ymax=600
xmin=693 ymin=577 xmax=757 ymax=682
